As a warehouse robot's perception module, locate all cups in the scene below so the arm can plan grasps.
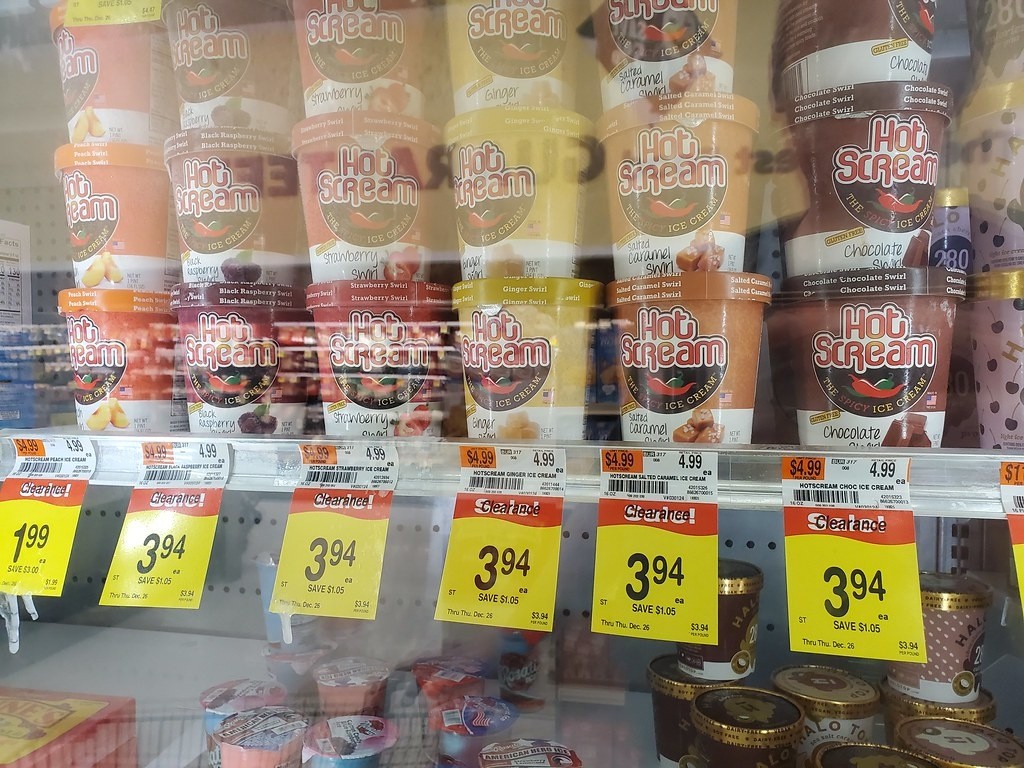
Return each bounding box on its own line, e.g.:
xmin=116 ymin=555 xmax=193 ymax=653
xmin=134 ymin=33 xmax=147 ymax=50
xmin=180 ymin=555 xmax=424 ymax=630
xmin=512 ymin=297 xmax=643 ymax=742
xmin=646 ymin=558 xmax=1024 ymax=768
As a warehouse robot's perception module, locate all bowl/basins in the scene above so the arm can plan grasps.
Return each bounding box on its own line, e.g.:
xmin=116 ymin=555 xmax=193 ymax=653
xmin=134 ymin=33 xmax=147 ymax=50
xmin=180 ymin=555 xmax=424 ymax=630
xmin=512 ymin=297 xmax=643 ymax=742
xmin=56 ymin=3 xmax=1021 ymax=451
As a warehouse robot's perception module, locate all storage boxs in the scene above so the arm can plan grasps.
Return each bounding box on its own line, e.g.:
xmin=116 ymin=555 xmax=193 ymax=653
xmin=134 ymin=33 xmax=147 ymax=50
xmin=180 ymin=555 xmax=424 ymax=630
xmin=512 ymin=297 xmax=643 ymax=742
xmin=0 ymin=683 xmax=144 ymax=768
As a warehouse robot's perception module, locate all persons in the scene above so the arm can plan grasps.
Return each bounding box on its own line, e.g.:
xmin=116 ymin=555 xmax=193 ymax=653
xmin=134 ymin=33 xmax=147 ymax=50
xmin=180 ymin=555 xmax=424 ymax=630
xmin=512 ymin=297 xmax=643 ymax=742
xmin=734 ymin=0 xmax=1024 ymax=463
